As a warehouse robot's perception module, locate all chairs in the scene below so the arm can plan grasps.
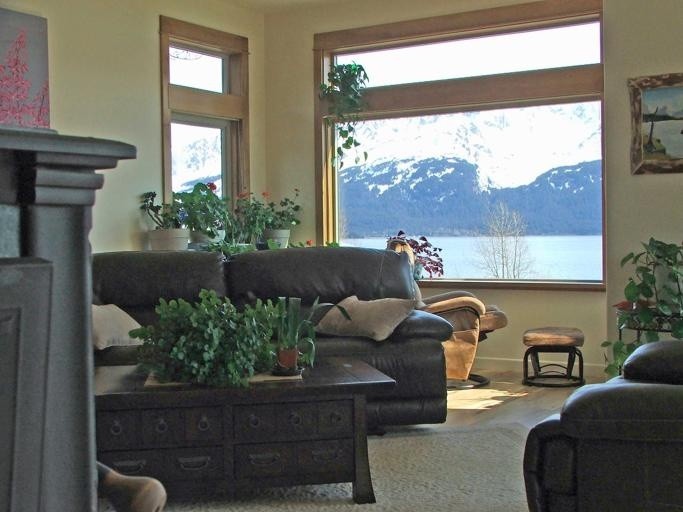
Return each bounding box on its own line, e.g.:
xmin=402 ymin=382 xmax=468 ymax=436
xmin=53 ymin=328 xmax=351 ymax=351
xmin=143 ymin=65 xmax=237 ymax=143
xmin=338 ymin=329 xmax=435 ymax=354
xmin=523 ymin=341 xmax=683 ymax=512
xmin=395 ymin=241 xmax=507 ymax=389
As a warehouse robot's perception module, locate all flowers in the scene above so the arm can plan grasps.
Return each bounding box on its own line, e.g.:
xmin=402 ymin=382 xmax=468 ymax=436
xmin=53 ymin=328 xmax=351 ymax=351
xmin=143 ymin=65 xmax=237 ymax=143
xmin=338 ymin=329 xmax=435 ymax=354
xmin=0 ymin=28 xmax=49 ymax=129
xmin=387 ymin=230 xmax=444 ymax=280
xmin=175 ymin=182 xmax=313 ymax=249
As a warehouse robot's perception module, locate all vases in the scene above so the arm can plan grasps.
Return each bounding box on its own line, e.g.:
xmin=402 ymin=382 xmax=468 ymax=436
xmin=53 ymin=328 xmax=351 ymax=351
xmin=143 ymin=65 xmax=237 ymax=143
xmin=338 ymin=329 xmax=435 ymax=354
xmin=413 ymin=262 xmax=423 ymax=280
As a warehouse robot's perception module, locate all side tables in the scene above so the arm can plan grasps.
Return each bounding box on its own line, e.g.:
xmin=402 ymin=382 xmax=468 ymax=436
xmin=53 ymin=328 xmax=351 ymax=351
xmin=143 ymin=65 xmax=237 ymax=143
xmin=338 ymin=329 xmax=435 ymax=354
xmin=612 ymin=299 xmax=683 ymax=375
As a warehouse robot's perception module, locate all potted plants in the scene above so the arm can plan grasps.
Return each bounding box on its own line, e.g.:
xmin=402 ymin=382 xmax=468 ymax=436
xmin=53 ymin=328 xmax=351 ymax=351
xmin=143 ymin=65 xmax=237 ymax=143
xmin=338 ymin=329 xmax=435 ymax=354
xmin=273 ymin=295 xmax=353 ymax=369
xmin=600 ymin=236 xmax=683 ymax=378
xmin=140 ymin=192 xmax=188 ymax=250
xmin=319 ymin=60 xmax=370 ymax=170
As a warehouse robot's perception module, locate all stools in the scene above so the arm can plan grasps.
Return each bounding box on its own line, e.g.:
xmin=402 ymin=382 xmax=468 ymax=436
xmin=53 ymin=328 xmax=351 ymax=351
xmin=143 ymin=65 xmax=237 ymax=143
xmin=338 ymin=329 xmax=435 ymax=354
xmin=522 ymin=327 xmax=585 ymax=387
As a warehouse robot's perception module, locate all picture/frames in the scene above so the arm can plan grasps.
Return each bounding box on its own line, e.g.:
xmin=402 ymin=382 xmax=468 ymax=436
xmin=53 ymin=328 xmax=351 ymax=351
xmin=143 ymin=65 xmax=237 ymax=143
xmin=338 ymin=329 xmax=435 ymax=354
xmin=627 ymin=73 xmax=683 ymax=175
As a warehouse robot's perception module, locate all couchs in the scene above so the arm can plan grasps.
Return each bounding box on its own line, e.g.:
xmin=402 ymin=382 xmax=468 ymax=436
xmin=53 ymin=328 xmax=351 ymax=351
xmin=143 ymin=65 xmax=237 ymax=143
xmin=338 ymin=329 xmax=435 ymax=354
xmin=91 ymin=247 xmax=454 ymax=435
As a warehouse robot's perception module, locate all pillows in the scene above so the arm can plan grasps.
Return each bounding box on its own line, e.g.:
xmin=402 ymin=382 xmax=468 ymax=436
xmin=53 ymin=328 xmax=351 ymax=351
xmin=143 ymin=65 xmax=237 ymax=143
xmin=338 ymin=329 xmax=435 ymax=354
xmin=91 ymin=303 xmax=154 ymax=351
xmin=314 ymin=295 xmax=417 ymax=342
